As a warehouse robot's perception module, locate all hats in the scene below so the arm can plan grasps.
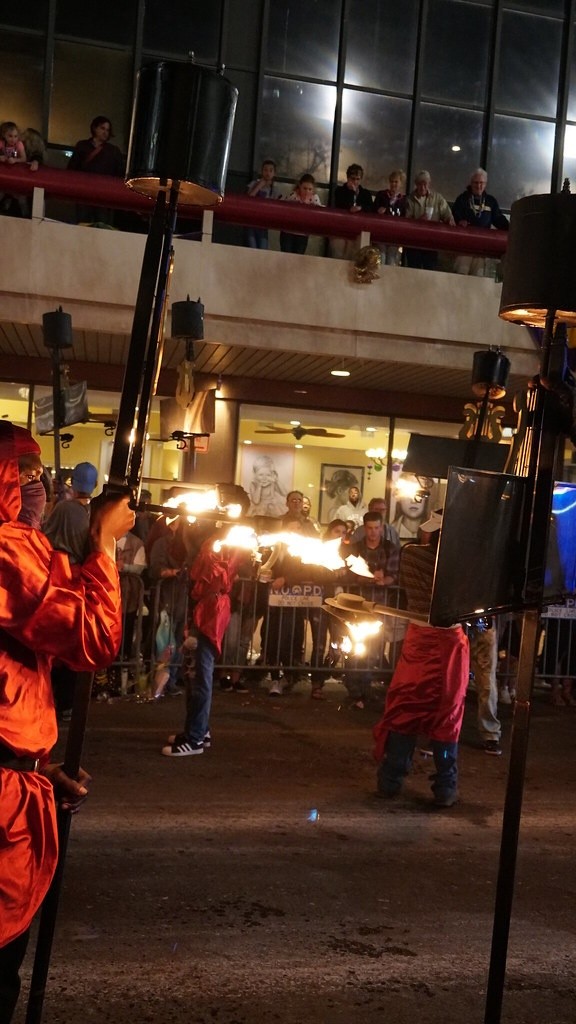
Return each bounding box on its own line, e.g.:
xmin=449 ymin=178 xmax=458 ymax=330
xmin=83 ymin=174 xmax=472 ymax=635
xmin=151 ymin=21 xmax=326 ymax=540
xmin=72 ymin=462 xmax=98 ymax=494
xmin=419 ymin=509 xmax=442 ymax=532
xmin=12 ymin=424 xmax=41 ymax=456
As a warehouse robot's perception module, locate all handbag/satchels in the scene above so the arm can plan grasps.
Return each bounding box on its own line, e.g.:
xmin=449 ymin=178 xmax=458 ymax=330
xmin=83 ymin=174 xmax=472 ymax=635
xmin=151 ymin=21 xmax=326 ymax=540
xmin=383 ymin=539 xmax=406 ymax=609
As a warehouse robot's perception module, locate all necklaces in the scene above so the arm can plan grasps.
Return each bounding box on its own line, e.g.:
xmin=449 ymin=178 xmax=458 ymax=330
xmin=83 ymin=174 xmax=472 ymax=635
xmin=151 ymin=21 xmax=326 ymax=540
xmin=470 ymin=196 xmax=485 ymax=212
xmin=75 ymin=497 xmax=92 ymax=498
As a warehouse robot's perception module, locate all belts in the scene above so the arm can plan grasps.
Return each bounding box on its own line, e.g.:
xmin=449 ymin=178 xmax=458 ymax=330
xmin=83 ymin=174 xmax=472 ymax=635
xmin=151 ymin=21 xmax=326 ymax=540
xmin=0 ymin=747 xmax=39 ymax=771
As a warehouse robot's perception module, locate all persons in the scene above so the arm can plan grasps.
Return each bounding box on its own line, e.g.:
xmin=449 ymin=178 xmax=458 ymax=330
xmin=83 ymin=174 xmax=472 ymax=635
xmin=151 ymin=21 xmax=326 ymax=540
xmin=162 ymin=484 xmax=250 ymax=756
xmin=329 ymin=164 xmax=509 ymax=276
xmin=280 ymin=174 xmax=323 ymax=255
xmin=245 ymin=160 xmax=283 ymax=249
xmin=370 ymin=510 xmax=470 ymax=807
xmin=213 ymin=490 xmax=408 ymax=709
xmin=497 ymin=618 xmax=576 ymax=706
xmin=91 ymin=490 xmax=207 ymax=703
xmin=41 ymin=462 xmax=98 ymax=720
xmin=66 ymin=115 xmax=122 ymax=230
xmin=0 ymin=419 xmax=135 ymax=1024
xmin=0 ymin=121 xmax=49 ymax=216
xmin=419 ymin=614 xmax=502 ymax=755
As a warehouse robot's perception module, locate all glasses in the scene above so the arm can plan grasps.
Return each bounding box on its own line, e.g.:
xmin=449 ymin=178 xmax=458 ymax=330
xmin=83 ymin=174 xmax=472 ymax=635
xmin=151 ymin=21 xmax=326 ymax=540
xmin=292 ymin=499 xmax=301 ymax=503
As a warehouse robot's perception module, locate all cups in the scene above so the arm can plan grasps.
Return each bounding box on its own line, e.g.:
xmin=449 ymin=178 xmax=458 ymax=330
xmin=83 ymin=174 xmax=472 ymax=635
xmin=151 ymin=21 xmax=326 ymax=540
xmin=426 ymin=207 xmax=433 ymax=220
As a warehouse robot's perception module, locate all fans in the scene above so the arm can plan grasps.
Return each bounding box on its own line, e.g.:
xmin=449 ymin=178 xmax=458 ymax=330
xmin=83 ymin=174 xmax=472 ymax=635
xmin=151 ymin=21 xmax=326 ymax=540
xmin=254 ymin=425 xmax=345 ymax=440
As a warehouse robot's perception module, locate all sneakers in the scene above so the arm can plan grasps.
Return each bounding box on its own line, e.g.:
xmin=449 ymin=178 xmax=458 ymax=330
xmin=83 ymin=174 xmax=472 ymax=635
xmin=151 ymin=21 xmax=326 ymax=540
xmin=161 ymin=736 xmax=204 ymax=757
xmin=169 ymin=728 xmax=211 ymax=747
xmin=498 ymin=685 xmax=530 ymax=706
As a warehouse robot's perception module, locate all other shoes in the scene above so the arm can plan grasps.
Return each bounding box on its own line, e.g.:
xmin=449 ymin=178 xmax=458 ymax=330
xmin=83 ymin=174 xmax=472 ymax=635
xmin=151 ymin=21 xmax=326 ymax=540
xmin=166 ymin=683 xmax=182 ymax=696
xmin=219 ymin=677 xmax=233 ymax=691
xmin=533 ymin=679 xmax=552 ymax=688
xmin=483 ymin=739 xmax=502 ymax=755
xmin=268 ymin=680 xmax=283 ymax=696
xmin=60 ymin=713 xmax=71 ymax=721
xmin=312 ymin=682 xmax=328 ymax=700
xmin=355 ymin=695 xmax=367 ymax=709
xmin=420 ymin=742 xmax=433 ymax=755
xmin=435 ymin=790 xmax=460 ymax=807
xmin=379 ymin=788 xmax=395 ymax=798
xmin=232 ymin=680 xmax=248 ymax=693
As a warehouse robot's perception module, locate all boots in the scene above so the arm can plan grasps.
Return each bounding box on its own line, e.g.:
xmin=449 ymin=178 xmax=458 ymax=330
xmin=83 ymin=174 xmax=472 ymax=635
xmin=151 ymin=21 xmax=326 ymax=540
xmin=550 ymin=679 xmax=565 ymax=706
xmin=562 ymin=678 xmax=576 ymax=706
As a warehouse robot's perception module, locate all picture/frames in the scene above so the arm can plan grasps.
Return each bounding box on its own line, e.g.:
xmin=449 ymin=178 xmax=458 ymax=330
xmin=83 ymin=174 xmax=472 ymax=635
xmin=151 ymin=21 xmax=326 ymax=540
xmin=97 ymin=438 xmax=152 ymax=493
xmin=317 ymin=462 xmax=365 ymax=527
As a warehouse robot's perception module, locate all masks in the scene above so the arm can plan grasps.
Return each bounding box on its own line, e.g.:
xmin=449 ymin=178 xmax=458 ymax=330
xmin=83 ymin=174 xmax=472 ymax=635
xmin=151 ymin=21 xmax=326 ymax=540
xmin=18 ymin=481 xmax=46 ymax=530
